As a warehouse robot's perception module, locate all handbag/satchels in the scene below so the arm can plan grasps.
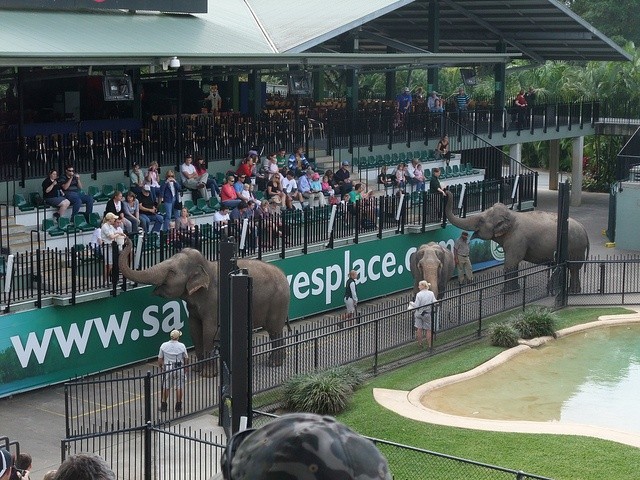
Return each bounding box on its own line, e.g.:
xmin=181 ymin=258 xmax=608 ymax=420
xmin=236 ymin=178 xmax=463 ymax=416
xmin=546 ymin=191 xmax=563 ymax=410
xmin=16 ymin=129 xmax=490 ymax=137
xmin=174 ymin=190 xmax=183 ymax=210
xmin=434 ymin=151 xmax=441 ymax=159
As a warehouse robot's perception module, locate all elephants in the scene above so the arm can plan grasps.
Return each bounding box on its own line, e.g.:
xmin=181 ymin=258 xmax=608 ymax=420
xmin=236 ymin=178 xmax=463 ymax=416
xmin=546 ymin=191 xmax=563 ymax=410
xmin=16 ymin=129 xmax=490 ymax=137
xmin=410 ymin=242 xmax=455 ymax=300
xmin=118 ymin=238 xmax=290 ymax=378
xmin=445 ymin=191 xmax=589 ymax=295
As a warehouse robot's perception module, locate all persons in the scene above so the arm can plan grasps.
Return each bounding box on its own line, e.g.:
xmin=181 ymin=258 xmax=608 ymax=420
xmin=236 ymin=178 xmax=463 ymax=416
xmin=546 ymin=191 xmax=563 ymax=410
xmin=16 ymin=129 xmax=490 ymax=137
xmin=42 ymin=170 xmax=70 ymax=221
xmin=90 ymin=223 xmax=102 ymax=258
xmin=394 ymin=86 xmax=413 ymax=112
xmin=414 ymin=87 xmax=425 ymax=103
xmin=268 ymin=158 xmax=278 ymax=179
xmin=217 ymin=199 xmax=292 ymax=250
xmin=115 ymin=217 xmax=127 ymax=254
xmin=101 ymin=212 xmax=126 ymax=282
xmin=416 ymin=165 xmax=425 ymax=181
xmin=407 ymin=160 xmax=429 ymax=191
xmin=177 ymin=208 xmax=195 ymax=248
xmin=276 ymin=149 xmax=288 ymax=175
xmin=515 ymin=88 xmax=529 ymax=106
xmin=427 ymin=91 xmax=440 ymax=109
xmin=0 ymin=449 xmax=16 ymax=480
xmin=234 ymin=173 xmax=249 ymax=203
xmin=312 ymin=173 xmax=322 ymax=194
xmin=221 ymin=176 xmax=241 ymax=209
xmin=145 ymin=162 xmax=162 ymax=202
xmin=179 ymin=156 xmax=209 ymax=201
xmin=324 ymin=174 xmax=336 ymax=200
xmin=243 ymin=184 xmax=251 ymax=203
xmin=267 ymin=173 xmax=287 ymax=209
xmin=252 ymin=154 xmax=264 ymax=186
xmin=43 ymin=469 xmax=55 ymax=480
xmin=165 ymin=220 xmax=182 ymax=249
xmin=342 ymin=183 xmax=378 ymax=230
xmin=54 ymin=452 xmax=119 ymax=480
xmin=123 ymin=192 xmax=147 ymax=231
xmin=249 ymin=186 xmax=261 ymax=204
xmin=379 ymin=168 xmax=393 ymax=184
xmin=452 ymin=230 xmax=479 ymax=287
xmin=195 ymin=155 xmax=221 ymax=201
xmin=130 ymin=162 xmax=149 ymax=197
xmin=60 ymin=165 xmax=94 ymax=222
xmin=281 ymin=169 xmax=304 ymax=209
xmin=396 ymin=165 xmax=407 ymax=191
xmin=236 ymin=158 xmax=252 ymax=186
xmin=138 ymin=186 xmax=161 ymax=233
xmin=15 ymin=453 xmax=33 ymax=480
xmin=158 ymin=330 xmax=188 ymax=413
xmin=161 ymin=170 xmax=180 ymax=230
xmin=454 ymin=87 xmax=473 ymax=112
xmin=105 ymin=190 xmax=132 ymax=234
xmin=336 ymin=269 xmax=361 ymax=328
xmin=288 ymin=146 xmax=315 ymax=176
xmin=430 ymin=168 xmax=447 ymax=196
xmin=406 ymin=280 xmax=439 ymax=349
xmin=261 ymin=152 xmax=274 ymax=184
xmin=430 ymin=100 xmax=444 ymax=113
xmin=297 ymin=169 xmax=326 ymax=205
xmin=438 ymin=135 xmax=451 ymax=166
xmin=392 ymin=163 xmax=409 ymax=192
xmin=325 ymin=170 xmax=339 ymax=189
xmin=338 ymin=159 xmax=356 ymax=198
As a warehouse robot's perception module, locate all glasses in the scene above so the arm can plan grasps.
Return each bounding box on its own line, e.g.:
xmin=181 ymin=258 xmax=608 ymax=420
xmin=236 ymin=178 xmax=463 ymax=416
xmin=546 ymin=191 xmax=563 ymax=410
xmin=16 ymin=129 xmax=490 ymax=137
xmin=168 ymin=175 xmax=174 ymax=178
xmin=230 ymin=180 xmax=234 ymax=182
xmin=241 ymin=177 xmax=245 ymax=178
xmin=276 ymin=177 xmax=280 ymax=179
xmin=68 ymin=170 xmax=74 ymax=172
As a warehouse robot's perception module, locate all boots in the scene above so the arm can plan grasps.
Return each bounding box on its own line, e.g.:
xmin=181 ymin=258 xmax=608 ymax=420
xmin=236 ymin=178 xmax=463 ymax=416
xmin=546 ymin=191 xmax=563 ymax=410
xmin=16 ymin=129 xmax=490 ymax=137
xmin=159 ymin=402 xmax=167 ymax=412
xmin=175 ymin=402 xmax=182 ymax=412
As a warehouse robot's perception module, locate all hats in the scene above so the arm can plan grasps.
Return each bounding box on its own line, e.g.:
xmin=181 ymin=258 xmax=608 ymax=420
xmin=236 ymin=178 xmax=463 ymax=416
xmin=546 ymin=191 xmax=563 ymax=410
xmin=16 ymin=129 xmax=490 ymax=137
xmin=306 ymin=170 xmax=314 ymax=174
xmin=0 ymin=450 xmax=11 ymax=478
xmin=314 ymin=172 xmax=320 ymax=179
xmin=269 ymin=198 xmax=281 ymax=204
xmin=131 ymin=162 xmax=137 ymax=167
xmin=342 ymin=160 xmax=349 ymax=166
xmin=431 ymin=91 xmax=438 ymax=95
xmin=106 ymin=212 xmax=119 ymax=219
xmin=184 ymin=154 xmax=193 ymax=158
xmin=404 ymin=87 xmax=409 ymax=92
xmin=142 ymin=184 xmax=151 ymax=192
xmin=170 ymin=330 xmax=182 ymax=340
xmin=461 ymin=232 xmax=468 ymax=237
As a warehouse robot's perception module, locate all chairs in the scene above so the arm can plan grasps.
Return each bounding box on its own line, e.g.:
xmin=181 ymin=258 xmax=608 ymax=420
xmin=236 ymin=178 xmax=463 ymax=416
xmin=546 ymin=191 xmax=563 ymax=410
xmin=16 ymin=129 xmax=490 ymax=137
xmin=381 ymin=162 xmax=473 ymax=189
xmin=190 ymin=157 xmax=363 ymax=238
xmin=352 ymin=148 xmax=481 ymax=169
xmin=13 ymin=180 xmax=190 ymax=237
xmin=402 ymin=178 xmax=497 ymax=207
